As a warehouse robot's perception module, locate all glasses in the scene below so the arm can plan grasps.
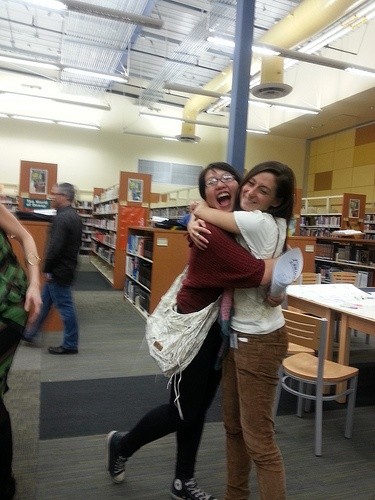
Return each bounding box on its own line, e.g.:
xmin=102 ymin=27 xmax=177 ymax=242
xmin=205 ymin=172 xmax=235 ymax=187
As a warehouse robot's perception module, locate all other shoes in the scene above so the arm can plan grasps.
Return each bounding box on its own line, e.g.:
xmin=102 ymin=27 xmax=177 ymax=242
xmin=48 ymin=345 xmax=78 ymax=355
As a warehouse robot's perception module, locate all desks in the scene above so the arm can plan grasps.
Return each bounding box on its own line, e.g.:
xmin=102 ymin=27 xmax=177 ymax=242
xmin=288 ymin=287 xmax=375 ymax=403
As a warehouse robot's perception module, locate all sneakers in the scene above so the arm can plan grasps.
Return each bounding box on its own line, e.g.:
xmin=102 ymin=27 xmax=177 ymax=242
xmin=106 ymin=431 xmax=125 ymax=483
xmin=168 ymin=476 xmax=219 ymax=500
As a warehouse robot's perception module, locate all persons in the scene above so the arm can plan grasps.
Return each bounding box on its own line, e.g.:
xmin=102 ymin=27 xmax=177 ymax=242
xmin=106 ymin=162 xmax=297 ymax=500
xmin=18 ymin=183 xmax=84 ymax=355
xmin=187 ymin=161 xmax=296 ymax=500
xmin=0 ymin=179 xmax=43 ymax=499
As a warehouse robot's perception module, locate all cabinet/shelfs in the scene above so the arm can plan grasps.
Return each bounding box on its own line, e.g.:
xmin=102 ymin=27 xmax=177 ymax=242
xmin=75 ymin=171 xmax=190 ymax=322
xmin=299 ymin=195 xmax=375 ymax=287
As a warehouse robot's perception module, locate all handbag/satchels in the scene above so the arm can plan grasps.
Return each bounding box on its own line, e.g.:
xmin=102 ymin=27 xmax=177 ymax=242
xmin=146 ymin=274 xmax=220 ymax=379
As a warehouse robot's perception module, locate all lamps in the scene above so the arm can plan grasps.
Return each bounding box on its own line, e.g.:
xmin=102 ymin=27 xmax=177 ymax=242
xmin=0 ymin=0 xmax=375 ymax=144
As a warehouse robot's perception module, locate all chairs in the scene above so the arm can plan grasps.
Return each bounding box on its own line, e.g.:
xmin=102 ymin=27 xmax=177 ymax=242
xmin=272 ymin=271 xmax=371 ymax=456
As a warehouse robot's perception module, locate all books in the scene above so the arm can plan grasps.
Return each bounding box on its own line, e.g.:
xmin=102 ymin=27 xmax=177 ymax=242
xmin=364 ymin=214 xmax=375 ymax=239
xmin=123 ymin=229 xmax=151 ymax=314
xmin=74 ymin=208 xmax=93 ymax=248
xmin=296 ymin=216 xmax=340 ymax=238
xmin=149 ymin=206 xmax=189 ymax=222
xmin=92 ymin=198 xmax=118 ymax=265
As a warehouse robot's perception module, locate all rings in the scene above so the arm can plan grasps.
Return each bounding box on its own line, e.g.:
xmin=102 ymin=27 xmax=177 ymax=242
xmin=196 ymin=234 xmax=199 ymax=237
